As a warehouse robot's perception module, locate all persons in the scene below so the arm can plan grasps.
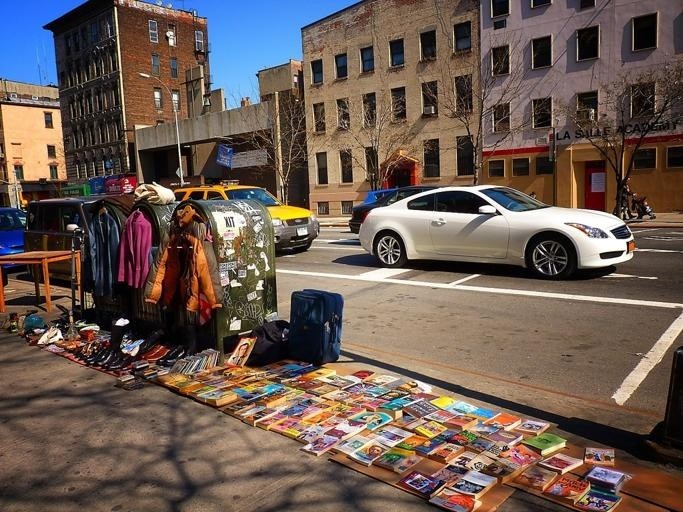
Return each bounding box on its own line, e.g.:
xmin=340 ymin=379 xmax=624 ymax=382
xmin=613 ymin=177 xmax=637 ymax=219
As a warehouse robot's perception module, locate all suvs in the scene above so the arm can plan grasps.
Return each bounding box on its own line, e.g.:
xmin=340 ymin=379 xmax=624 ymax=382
xmin=173 ymin=179 xmax=320 ymax=255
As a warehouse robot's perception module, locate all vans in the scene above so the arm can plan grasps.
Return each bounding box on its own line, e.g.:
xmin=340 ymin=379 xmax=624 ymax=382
xmin=21 ymin=193 xmax=124 ymax=286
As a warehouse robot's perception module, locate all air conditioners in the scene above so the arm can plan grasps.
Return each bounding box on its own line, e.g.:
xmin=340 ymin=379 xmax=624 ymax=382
xmin=337 ymin=120 xmax=346 ymax=129
xmin=573 ymin=107 xmax=595 ymax=126
xmin=421 ymin=105 xmax=434 ymax=114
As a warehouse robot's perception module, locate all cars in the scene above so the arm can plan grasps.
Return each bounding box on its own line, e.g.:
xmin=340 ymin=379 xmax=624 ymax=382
xmin=0 ymin=206 xmax=29 ymax=269
xmin=348 ymin=185 xmax=449 ymax=234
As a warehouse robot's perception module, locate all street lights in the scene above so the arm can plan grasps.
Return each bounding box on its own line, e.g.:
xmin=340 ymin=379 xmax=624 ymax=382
xmin=135 ymin=70 xmax=184 ymax=190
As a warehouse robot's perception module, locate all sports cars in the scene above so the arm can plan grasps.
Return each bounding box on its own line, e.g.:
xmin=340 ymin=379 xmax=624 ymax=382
xmin=355 ymin=185 xmax=636 ymax=282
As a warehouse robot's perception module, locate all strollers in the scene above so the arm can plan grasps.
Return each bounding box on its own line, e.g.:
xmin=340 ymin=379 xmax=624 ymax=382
xmin=632 ymin=192 xmax=656 ymax=220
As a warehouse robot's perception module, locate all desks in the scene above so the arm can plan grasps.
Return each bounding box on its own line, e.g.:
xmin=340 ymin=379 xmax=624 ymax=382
xmin=0 ymin=250 xmax=81 ymax=319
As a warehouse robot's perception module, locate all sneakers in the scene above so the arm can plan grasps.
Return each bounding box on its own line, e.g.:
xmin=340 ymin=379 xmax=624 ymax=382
xmin=54 ymin=322 xmax=187 ymax=370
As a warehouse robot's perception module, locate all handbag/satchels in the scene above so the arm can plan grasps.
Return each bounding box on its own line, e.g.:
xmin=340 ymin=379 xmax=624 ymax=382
xmin=1 ymin=311 xmax=63 ymax=345
xmin=246 ymin=320 xmax=290 ymax=368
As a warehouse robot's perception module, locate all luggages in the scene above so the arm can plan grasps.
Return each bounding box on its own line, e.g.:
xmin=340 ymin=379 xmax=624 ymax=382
xmin=286 ymin=288 xmax=345 ymax=367
